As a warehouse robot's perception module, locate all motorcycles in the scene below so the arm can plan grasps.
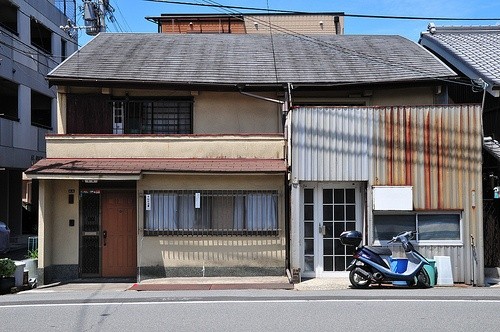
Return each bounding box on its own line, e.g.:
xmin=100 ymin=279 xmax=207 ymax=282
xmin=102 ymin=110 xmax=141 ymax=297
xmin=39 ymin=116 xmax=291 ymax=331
xmin=339 ymin=227 xmax=432 ymax=289
